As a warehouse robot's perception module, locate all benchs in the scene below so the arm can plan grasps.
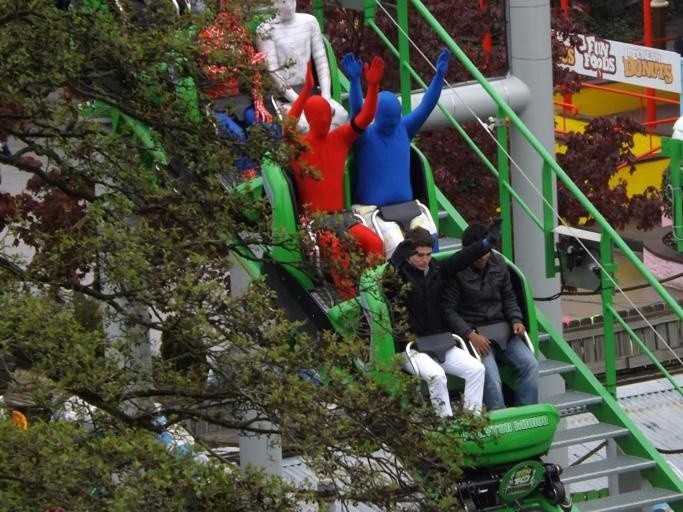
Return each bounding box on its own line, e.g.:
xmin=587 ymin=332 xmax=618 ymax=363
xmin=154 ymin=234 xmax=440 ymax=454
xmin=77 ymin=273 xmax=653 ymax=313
xmin=159 ymin=14 xmax=343 ymax=171
xmin=259 ymin=137 xmax=440 ymax=284
xmin=361 ymin=247 xmax=537 ymax=412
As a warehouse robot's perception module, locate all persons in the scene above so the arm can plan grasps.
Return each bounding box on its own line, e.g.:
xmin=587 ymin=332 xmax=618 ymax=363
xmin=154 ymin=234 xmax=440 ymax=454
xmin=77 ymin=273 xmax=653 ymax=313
xmin=258 ymin=0 xmax=348 ymax=137
xmin=381 ymin=216 xmax=504 ymax=422
xmin=195 ymin=0 xmax=282 ymax=188
xmin=341 ymin=46 xmax=450 ymax=264
xmin=278 ymin=55 xmax=384 ymax=304
xmin=437 ymin=220 xmax=539 ymax=412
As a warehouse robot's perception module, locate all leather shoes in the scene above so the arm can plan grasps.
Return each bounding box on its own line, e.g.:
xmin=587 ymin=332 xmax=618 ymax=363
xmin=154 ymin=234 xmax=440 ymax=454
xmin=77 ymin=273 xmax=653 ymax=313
xmin=387 ymin=239 xmax=417 ymax=267
xmin=487 ymin=218 xmax=503 ymax=247
